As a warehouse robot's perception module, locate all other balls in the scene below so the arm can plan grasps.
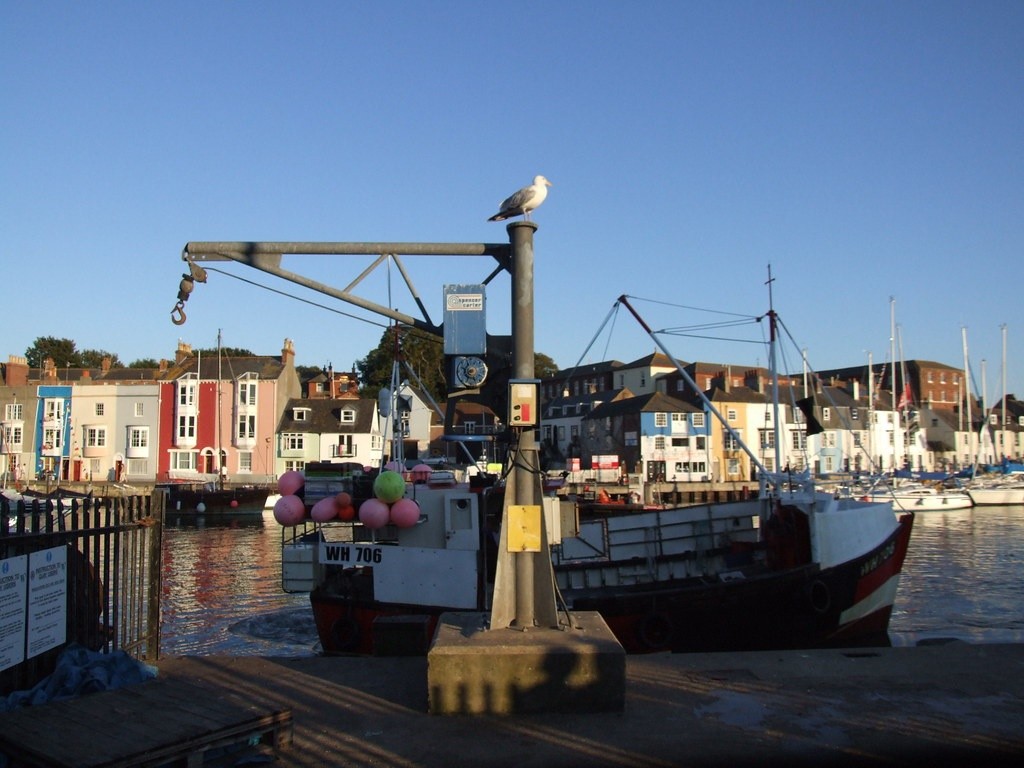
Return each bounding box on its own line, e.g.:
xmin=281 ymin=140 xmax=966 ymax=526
xmin=271 ymin=463 xmax=433 ymax=530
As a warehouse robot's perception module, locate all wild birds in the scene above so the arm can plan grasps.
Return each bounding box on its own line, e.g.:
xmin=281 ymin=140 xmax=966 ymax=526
xmin=486 ymin=174 xmax=552 ymax=223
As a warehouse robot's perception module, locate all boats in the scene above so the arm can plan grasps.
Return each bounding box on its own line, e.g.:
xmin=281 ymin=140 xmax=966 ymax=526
xmin=282 ymin=293 xmax=915 ymax=653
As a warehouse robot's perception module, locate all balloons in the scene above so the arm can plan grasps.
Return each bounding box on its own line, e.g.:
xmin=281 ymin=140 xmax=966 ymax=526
xmin=359 ymin=462 xmax=432 ymax=529
xmin=312 ymin=493 xmax=354 ymax=523
xmin=273 ymin=471 xmax=305 ymax=527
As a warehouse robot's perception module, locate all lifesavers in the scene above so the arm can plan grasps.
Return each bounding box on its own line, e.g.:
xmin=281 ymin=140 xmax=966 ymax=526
xmin=629 ymin=490 xmax=641 ymax=502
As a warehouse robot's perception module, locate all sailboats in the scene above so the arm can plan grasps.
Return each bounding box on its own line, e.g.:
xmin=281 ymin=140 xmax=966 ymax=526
xmin=154 ymin=329 xmax=271 ymax=516
xmin=0 ymin=404 xmax=103 ymax=510
xmin=763 ymin=296 xmax=1024 ymax=510
